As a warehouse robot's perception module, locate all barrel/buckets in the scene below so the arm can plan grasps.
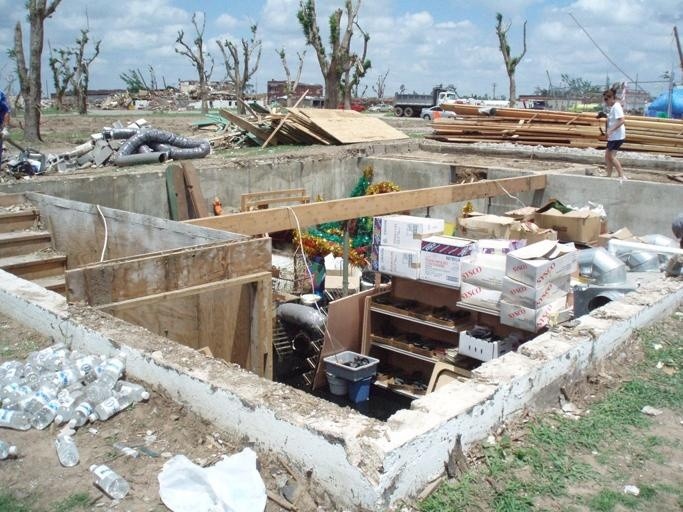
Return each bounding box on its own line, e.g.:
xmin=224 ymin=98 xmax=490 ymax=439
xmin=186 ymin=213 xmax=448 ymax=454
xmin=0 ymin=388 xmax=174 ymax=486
xmin=347 ymin=376 xmax=371 ymax=403
xmin=304 ymin=255 xmax=326 ymax=290
xmin=324 ymin=372 xmax=346 ymax=396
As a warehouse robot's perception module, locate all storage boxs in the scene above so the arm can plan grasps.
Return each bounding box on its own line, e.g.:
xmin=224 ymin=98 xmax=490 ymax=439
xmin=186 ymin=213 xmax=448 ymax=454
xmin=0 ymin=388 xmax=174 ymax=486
xmin=371 ymin=197 xmax=606 ymax=335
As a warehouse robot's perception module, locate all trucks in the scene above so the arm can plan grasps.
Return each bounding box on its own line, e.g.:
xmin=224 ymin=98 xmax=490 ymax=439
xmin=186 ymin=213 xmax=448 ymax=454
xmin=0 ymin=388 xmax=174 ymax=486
xmin=392 ymin=91 xmax=462 ymax=117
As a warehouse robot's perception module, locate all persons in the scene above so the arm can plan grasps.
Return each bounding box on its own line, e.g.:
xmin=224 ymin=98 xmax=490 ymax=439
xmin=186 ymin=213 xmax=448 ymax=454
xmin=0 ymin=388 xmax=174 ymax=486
xmin=0 ymin=91 xmax=10 ymax=170
xmin=597 ymin=89 xmax=626 ymax=179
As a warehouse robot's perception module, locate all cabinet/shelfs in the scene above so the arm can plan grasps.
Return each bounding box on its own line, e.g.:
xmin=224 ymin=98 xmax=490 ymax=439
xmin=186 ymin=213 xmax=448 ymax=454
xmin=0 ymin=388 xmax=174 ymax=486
xmin=364 ymin=290 xmax=471 ymax=401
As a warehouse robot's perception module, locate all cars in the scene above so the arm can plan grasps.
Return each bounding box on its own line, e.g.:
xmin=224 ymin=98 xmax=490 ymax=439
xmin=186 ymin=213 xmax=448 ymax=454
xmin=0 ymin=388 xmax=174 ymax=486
xmin=420 ymin=106 xmax=457 ymax=121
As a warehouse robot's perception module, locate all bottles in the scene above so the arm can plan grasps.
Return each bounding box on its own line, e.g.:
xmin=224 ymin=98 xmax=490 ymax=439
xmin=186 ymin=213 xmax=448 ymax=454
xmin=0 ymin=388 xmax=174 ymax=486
xmin=88 ymin=463 xmax=130 ymax=502
xmin=0 ymin=439 xmax=21 ymax=462
xmin=55 ymin=431 xmax=80 ymax=468
xmin=112 ymin=441 xmax=140 ymax=459
xmin=0 ymin=342 xmax=150 ymax=432
